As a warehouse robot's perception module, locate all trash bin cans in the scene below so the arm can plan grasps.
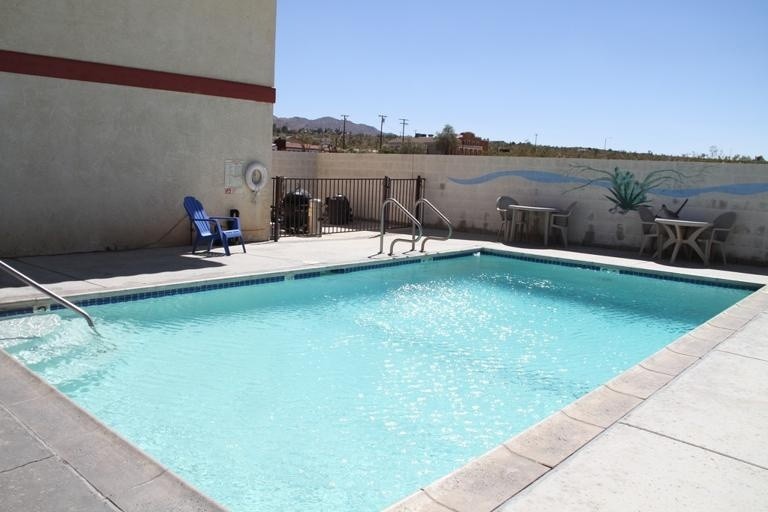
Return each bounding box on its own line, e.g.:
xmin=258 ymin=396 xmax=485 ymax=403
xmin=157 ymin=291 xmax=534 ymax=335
xmin=308 ymin=199 xmax=321 ymax=236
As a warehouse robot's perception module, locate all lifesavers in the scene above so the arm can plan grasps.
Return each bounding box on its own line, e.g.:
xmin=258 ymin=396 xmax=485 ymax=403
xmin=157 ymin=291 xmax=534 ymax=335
xmin=246 ymin=163 xmax=267 ymax=192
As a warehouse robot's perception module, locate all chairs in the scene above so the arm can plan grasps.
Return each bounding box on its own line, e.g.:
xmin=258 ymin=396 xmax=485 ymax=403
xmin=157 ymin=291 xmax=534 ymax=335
xmin=638 ymin=205 xmax=738 ymax=267
xmin=183 ymin=196 xmax=246 ymax=256
xmin=495 ymin=195 xmax=578 ymax=248
